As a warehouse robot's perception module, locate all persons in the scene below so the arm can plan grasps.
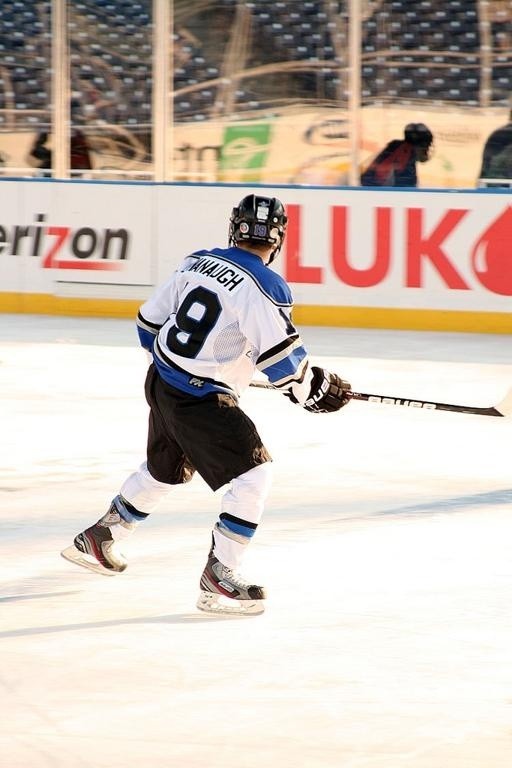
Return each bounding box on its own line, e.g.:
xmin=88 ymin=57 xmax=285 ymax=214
xmin=72 ymin=195 xmax=354 ymax=604
xmin=29 ymin=98 xmax=92 ymax=178
xmin=477 ymin=110 xmax=512 ymax=190
xmin=358 ymin=123 xmax=435 ymax=188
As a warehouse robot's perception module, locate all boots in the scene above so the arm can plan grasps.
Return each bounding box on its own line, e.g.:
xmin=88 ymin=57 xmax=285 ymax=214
xmin=73 ymin=498 xmax=140 ymax=574
xmin=197 ymin=522 xmax=266 ymax=601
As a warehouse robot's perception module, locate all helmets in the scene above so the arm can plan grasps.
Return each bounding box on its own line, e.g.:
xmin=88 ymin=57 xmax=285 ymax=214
xmin=231 ymin=194 xmax=288 ymax=245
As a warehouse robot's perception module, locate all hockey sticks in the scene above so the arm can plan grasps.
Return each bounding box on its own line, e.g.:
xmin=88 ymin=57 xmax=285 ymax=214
xmin=249 ymin=381 xmax=511 ymax=417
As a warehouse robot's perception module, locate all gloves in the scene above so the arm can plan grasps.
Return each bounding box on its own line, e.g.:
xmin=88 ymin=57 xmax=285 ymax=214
xmin=289 ymin=366 xmax=352 ymax=414
